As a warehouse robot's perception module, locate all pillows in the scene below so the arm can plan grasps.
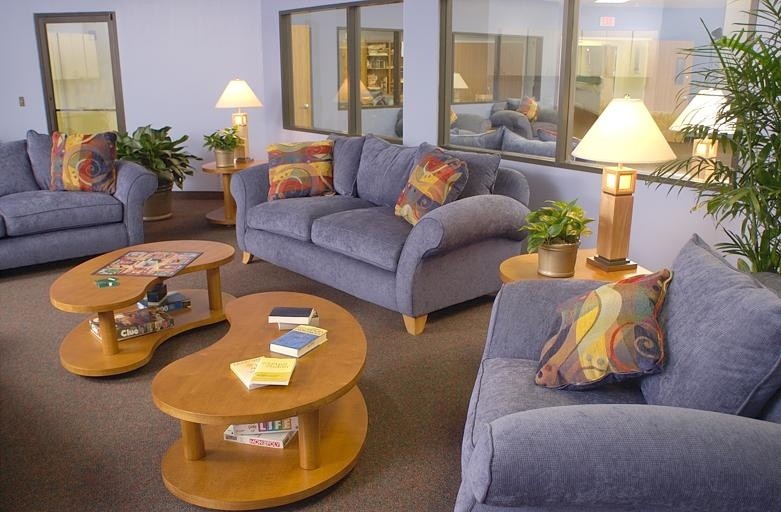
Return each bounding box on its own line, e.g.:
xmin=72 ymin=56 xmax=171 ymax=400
xmin=264 ymin=139 xmax=336 ymax=202
xmin=393 ymin=143 xmax=470 ymax=228
xmin=46 ymin=129 xmax=120 ymax=198
xmin=533 ymin=267 xmax=678 ymax=393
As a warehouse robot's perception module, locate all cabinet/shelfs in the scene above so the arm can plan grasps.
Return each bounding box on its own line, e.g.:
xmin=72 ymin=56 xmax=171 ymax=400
xmin=362 ymin=37 xmax=402 ymax=105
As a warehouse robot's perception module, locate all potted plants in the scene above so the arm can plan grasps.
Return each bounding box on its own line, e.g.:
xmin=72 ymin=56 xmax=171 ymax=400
xmin=202 ymin=122 xmax=245 ymax=169
xmin=516 ymin=197 xmax=598 ymax=277
xmin=116 ymin=121 xmax=203 ymax=224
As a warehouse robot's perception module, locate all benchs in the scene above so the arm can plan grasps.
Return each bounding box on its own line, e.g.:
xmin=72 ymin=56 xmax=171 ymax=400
xmin=395 ymin=92 xmax=581 ymax=161
xmin=450 ymin=232 xmax=781 ymax=512
xmin=228 ymin=131 xmax=535 ymax=337
xmin=0 ymin=125 xmax=160 ymax=275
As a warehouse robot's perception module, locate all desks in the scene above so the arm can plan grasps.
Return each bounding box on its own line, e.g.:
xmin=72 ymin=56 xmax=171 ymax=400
xmin=199 ymin=157 xmax=269 ymax=226
xmin=148 ymin=288 xmax=373 ymax=510
xmin=48 ymin=239 xmax=240 ymax=380
xmin=494 ymin=244 xmax=652 ymax=286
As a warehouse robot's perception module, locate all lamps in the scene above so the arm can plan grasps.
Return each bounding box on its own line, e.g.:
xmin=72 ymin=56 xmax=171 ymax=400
xmin=668 ymin=85 xmax=739 ymax=180
xmin=569 ymin=93 xmax=678 ymax=272
xmin=451 ymin=71 xmax=467 ymax=101
xmin=214 ymin=74 xmax=263 ymax=164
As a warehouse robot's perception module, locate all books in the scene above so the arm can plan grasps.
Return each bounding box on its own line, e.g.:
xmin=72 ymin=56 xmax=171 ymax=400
xmin=269 ymin=323 xmax=329 ymax=360
xmin=136 ymin=291 xmax=194 ymax=311
xmin=267 ymin=305 xmax=314 ymax=324
xmin=248 ymin=356 xmax=297 ymax=386
xmin=88 ymin=305 xmax=175 ymax=340
xmin=223 ymin=423 xmax=299 ymax=451
xmin=372 ymin=58 xmax=388 ymax=68
xmin=228 ymin=356 xmax=270 ymax=391
xmin=233 ymin=415 xmax=300 ymax=434
xmin=276 ymin=308 xmax=321 ymax=331
